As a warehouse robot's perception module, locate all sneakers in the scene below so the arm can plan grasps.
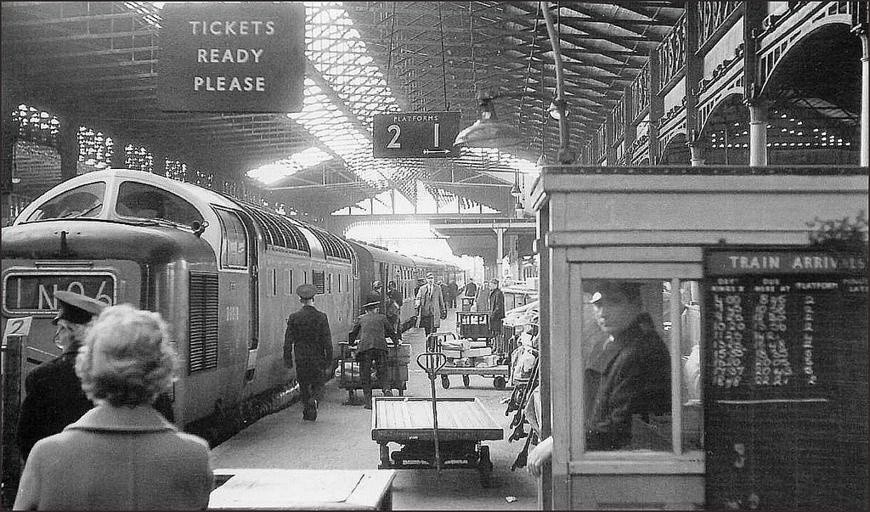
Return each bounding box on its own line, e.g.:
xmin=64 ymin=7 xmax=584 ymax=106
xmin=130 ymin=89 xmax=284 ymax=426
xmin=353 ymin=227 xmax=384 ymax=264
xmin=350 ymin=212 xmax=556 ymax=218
xmin=308 ymin=398 xmax=318 ymax=420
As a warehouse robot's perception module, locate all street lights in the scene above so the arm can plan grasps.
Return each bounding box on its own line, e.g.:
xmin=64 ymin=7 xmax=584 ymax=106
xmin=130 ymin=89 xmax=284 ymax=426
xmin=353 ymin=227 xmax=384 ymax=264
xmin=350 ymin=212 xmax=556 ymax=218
xmin=447 ymin=79 xmax=582 ymax=166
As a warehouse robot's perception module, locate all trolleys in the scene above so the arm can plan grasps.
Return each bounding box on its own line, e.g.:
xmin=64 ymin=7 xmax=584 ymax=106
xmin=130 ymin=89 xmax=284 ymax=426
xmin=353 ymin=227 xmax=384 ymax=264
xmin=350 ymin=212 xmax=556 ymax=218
xmin=365 ymin=348 xmax=508 ymax=489
xmin=453 ymin=311 xmax=494 ymax=347
xmin=339 ymin=334 xmax=406 ymax=404
xmin=426 ymin=329 xmax=511 ymax=392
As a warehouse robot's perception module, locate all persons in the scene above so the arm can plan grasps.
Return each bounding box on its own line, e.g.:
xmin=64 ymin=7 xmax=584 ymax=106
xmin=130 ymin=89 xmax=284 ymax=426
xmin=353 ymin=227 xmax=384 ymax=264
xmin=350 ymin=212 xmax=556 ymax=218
xmin=370 ymin=272 xmax=504 ymax=358
xmin=350 ymin=294 xmax=401 ymax=412
xmin=283 ymin=284 xmax=336 ymax=423
xmin=15 ymin=291 xmax=105 ymax=469
xmin=11 ymin=306 xmax=212 ymax=512
xmin=524 ymin=277 xmax=671 ymax=481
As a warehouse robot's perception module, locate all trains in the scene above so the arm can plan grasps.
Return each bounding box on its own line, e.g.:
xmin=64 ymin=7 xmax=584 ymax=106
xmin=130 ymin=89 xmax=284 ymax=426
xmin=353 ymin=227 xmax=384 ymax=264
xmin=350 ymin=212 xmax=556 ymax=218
xmin=0 ymin=167 xmax=470 ymax=465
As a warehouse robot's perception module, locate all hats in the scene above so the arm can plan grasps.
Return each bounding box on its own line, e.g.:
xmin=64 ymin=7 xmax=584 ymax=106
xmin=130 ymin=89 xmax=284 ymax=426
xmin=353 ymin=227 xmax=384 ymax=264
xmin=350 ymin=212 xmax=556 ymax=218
xmin=584 ymin=282 xmax=647 ymax=304
xmin=52 ymin=291 xmax=105 ymax=325
xmin=373 ymin=280 xmax=383 ymax=287
xmin=362 ymin=302 xmax=380 ymax=310
xmin=296 ymin=284 xmax=318 ymax=300
